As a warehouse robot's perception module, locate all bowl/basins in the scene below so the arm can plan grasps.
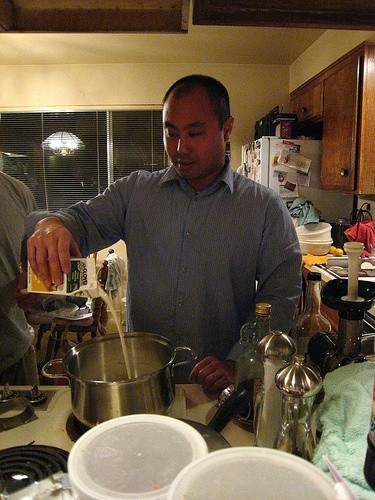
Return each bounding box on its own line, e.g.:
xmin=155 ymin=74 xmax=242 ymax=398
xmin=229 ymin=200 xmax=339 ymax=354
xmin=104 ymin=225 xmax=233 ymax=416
xmin=295 ymin=222 xmax=333 ymax=255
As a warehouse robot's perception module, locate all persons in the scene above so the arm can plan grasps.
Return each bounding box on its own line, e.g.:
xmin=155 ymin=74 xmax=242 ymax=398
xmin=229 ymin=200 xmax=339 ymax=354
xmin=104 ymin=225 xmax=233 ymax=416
xmin=26 ymin=74 xmax=303 ymax=392
xmin=0 ymin=172 xmax=39 ymax=386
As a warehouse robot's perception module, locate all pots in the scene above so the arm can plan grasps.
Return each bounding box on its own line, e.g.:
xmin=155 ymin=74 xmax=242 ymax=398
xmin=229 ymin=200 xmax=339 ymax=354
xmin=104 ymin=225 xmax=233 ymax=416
xmin=41 ymin=332 xmax=196 ymax=428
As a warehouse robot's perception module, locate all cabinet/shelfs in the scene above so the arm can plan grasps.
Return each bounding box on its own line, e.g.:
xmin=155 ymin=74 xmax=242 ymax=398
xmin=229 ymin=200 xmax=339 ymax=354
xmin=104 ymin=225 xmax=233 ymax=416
xmin=290 ymin=42 xmax=375 ymax=197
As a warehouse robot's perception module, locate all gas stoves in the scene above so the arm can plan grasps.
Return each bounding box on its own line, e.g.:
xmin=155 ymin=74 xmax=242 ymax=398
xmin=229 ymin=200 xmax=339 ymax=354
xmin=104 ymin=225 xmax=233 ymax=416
xmin=0 ymin=384 xmax=187 ymax=500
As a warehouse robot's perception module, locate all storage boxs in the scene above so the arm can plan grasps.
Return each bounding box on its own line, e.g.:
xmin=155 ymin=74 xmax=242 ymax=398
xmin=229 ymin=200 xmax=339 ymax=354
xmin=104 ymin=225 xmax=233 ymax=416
xmin=255 ymin=106 xmax=297 ymax=140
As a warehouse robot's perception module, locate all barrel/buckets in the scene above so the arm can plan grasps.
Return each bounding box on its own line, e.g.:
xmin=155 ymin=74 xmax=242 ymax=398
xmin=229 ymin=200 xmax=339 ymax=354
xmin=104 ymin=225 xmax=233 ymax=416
xmin=169 ymin=446 xmax=342 ymax=500
xmin=67 ymin=414 xmax=209 ymax=500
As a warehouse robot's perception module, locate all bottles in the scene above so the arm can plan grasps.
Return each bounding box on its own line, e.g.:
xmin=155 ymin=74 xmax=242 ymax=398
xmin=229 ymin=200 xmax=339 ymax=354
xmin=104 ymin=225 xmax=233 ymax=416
xmin=290 ymin=273 xmax=332 ymax=338
xmin=236 ymin=303 xmax=276 ymax=432
xmin=273 ymin=355 xmax=324 ymax=463
xmin=254 ymin=329 xmax=299 ymax=448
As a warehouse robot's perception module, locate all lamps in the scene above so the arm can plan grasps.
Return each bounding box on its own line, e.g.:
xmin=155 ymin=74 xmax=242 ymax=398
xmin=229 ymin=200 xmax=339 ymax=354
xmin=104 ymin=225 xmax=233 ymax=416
xmin=41 ymin=117 xmax=86 ymax=158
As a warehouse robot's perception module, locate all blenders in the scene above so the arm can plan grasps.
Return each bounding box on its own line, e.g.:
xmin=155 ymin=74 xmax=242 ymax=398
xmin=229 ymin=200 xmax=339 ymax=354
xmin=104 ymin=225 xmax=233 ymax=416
xmin=308 ymin=241 xmax=375 ymax=405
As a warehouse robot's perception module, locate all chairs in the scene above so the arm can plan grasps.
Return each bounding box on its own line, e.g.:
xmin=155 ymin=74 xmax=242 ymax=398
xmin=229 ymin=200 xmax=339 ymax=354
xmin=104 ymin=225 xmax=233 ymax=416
xmin=26 ymin=251 xmax=115 ymax=360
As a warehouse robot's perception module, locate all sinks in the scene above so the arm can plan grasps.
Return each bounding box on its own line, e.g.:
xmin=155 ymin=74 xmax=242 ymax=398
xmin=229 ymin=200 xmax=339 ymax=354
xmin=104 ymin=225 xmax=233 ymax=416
xmin=317 ymin=257 xmax=375 ymax=269
xmin=326 ymin=269 xmax=375 ymax=279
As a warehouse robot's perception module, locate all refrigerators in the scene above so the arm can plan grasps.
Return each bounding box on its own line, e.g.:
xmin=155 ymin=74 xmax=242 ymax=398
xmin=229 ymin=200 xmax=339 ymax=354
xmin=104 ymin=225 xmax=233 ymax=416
xmin=241 ymin=136 xmax=354 ymax=222
